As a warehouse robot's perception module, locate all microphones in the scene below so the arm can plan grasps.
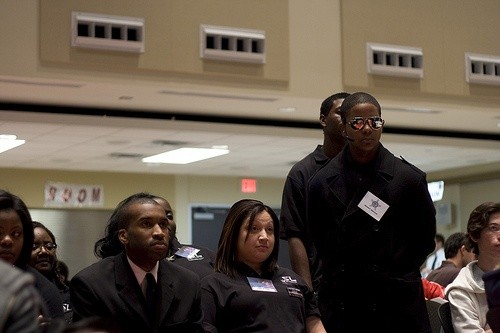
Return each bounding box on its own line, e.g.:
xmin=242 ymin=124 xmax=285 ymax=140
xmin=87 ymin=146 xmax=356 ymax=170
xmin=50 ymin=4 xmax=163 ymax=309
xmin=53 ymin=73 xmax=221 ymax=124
xmin=344 ymin=136 xmax=355 ymax=141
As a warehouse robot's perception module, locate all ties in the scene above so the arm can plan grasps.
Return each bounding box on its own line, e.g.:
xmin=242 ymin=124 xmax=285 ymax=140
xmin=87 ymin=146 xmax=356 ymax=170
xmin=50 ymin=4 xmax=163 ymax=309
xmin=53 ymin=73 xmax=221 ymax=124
xmin=145 ymin=273 xmax=158 ymax=322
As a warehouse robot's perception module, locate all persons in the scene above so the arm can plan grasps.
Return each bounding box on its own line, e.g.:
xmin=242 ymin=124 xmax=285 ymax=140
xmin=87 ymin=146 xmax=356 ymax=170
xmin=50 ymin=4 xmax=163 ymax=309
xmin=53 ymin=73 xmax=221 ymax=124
xmin=0 ymin=91 xmax=500 ymax=333
xmin=69 ymin=192 xmax=206 ymax=333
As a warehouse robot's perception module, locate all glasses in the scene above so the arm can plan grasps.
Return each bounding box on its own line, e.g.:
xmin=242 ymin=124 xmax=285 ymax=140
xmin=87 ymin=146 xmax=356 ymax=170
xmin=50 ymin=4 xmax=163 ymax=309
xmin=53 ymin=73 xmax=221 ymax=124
xmin=32 ymin=243 xmax=56 ymax=249
xmin=346 ymin=117 xmax=383 ymax=131
